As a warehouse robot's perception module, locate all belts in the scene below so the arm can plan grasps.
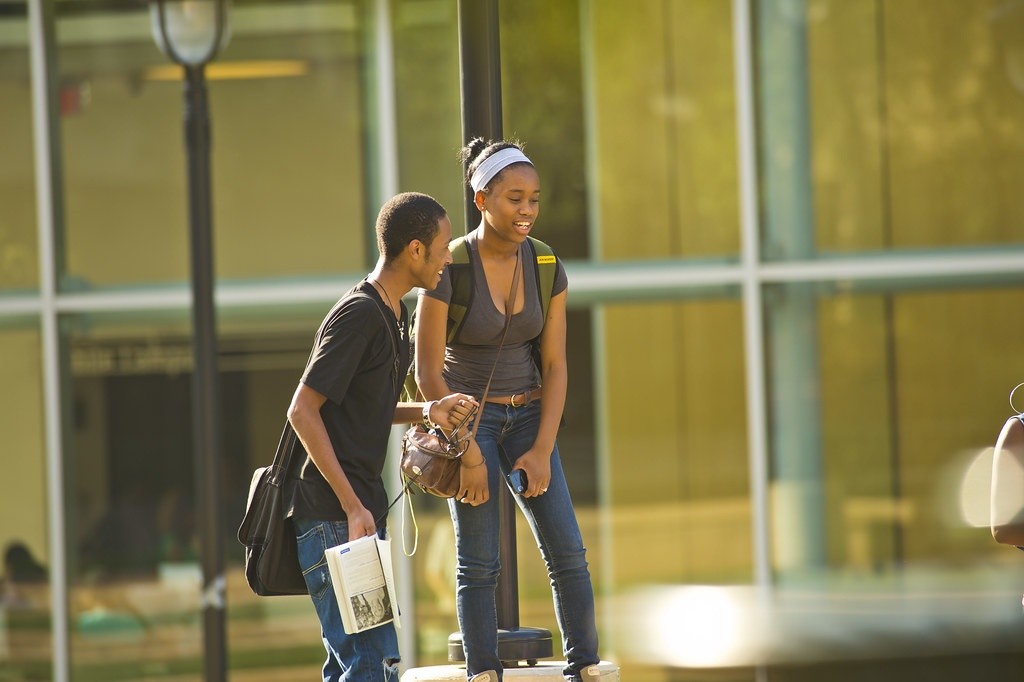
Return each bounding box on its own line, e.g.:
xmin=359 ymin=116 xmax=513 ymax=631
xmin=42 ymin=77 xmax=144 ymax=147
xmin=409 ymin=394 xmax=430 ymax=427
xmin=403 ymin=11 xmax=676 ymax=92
xmin=485 ymin=385 xmax=543 ymax=408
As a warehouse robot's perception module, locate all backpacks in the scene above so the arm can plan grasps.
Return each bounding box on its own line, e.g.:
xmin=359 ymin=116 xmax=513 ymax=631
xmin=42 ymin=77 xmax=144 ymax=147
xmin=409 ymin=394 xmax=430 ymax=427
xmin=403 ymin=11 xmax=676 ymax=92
xmin=399 ymin=233 xmax=560 ymax=403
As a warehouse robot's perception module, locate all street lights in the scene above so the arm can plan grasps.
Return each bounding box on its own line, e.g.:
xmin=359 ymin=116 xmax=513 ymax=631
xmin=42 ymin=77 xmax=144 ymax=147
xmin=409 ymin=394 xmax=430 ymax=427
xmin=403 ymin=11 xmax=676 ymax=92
xmin=148 ymin=0 xmax=234 ymax=679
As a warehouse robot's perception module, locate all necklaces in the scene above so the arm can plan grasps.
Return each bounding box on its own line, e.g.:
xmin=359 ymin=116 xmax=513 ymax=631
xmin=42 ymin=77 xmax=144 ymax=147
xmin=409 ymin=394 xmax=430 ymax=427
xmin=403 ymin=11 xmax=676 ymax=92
xmin=368 ymin=273 xmax=406 ymax=342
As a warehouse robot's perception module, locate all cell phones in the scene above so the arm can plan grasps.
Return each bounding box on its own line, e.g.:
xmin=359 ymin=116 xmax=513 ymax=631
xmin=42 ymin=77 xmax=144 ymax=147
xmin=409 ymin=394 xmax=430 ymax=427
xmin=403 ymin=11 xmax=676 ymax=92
xmin=508 ymin=468 xmax=528 ymax=494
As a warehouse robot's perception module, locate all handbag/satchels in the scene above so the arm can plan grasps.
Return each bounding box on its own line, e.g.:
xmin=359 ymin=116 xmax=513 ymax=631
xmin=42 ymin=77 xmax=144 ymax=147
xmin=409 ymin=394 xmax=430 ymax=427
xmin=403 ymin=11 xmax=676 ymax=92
xmin=237 ymin=467 xmax=310 ymax=597
xmin=398 ymin=422 xmax=470 ymax=499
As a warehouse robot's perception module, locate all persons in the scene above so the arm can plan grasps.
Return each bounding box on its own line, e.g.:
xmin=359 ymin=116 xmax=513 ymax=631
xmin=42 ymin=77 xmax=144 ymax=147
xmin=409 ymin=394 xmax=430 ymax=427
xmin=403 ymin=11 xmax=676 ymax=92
xmin=272 ymin=190 xmax=451 ymax=682
xmin=410 ymin=135 xmax=620 ymax=682
